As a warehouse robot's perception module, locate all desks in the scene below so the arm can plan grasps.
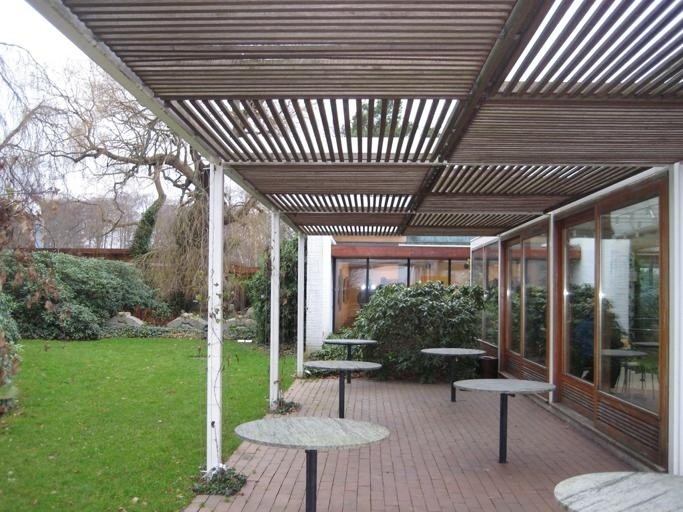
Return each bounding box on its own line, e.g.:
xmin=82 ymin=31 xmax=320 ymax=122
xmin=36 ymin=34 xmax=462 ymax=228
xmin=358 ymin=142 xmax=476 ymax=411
xmin=453 ymin=379 xmax=556 ymax=464
xmin=324 ymin=339 xmax=377 ymax=384
xmin=234 ymin=416 xmax=392 ymax=511
xmin=554 ymin=471 xmax=682 ymax=511
xmin=302 ymin=360 xmax=383 ymax=419
xmin=420 ymin=347 xmax=487 ymax=403
